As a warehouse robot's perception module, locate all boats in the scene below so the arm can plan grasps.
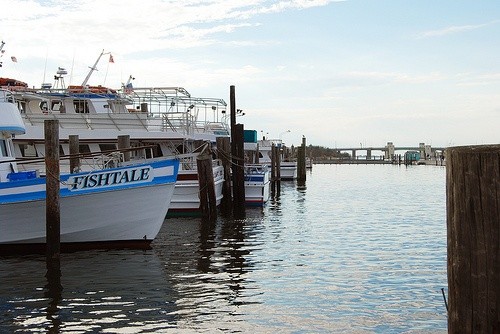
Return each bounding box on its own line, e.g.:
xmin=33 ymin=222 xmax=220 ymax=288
xmin=0 ymin=50 xmax=314 ymax=255
